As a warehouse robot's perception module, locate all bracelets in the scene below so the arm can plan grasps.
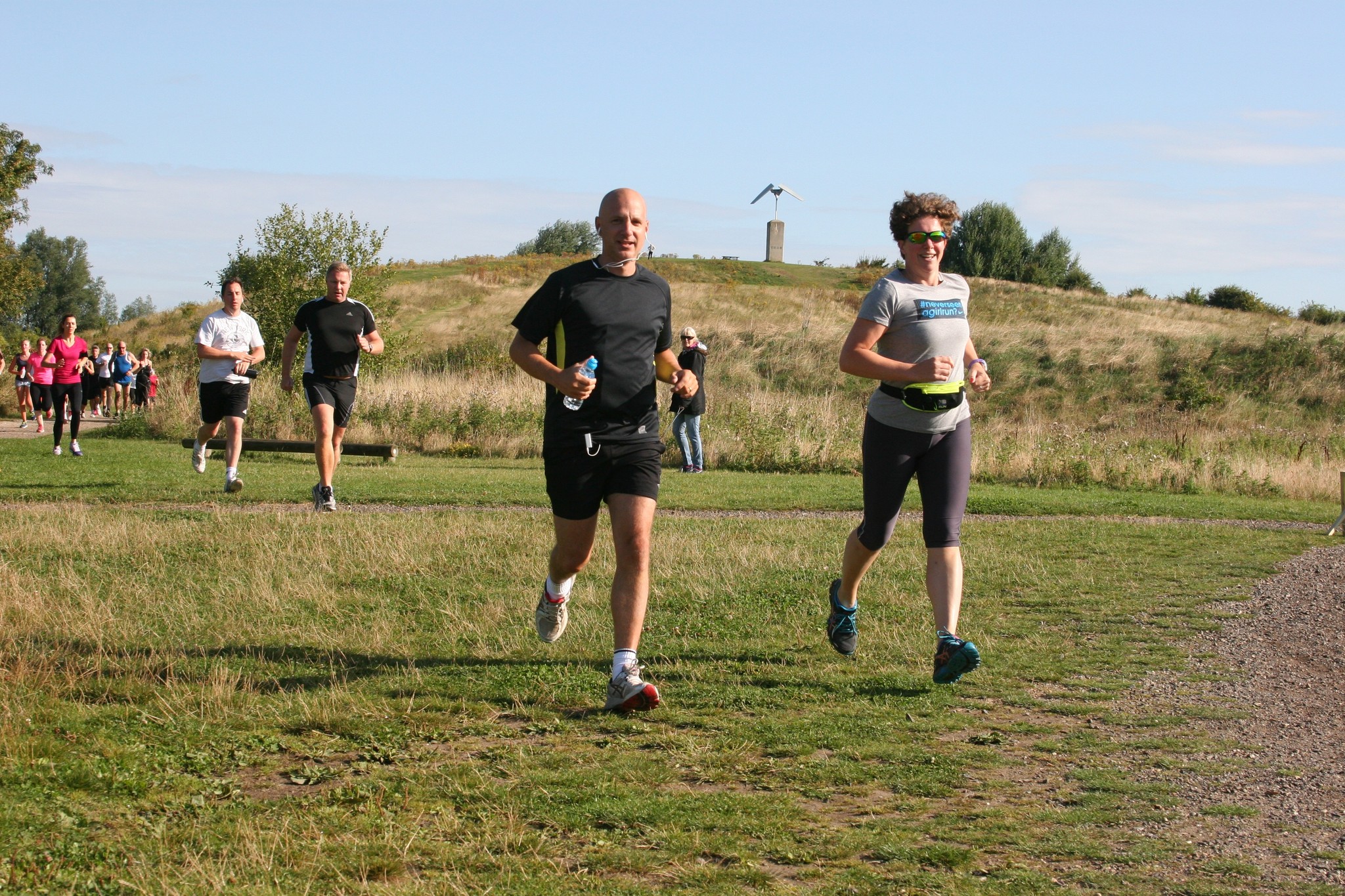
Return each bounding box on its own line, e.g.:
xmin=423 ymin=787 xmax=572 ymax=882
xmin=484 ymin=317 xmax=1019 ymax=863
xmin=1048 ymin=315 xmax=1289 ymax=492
xmin=249 ymin=360 xmax=252 ymax=367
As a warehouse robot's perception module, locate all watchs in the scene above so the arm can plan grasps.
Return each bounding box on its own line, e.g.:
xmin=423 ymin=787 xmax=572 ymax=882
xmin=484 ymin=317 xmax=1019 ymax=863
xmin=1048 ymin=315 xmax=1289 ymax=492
xmin=130 ymin=369 xmax=133 ymax=373
xmin=968 ymin=359 xmax=987 ymax=371
xmin=365 ymin=343 xmax=373 ymax=354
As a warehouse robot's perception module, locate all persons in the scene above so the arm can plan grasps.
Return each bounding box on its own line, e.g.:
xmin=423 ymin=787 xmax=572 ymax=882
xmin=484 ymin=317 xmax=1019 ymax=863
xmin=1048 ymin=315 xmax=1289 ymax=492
xmin=668 ymin=326 xmax=708 ymax=474
xmin=108 ymin=341 xmax=140 ymax=420
xmin=648 ymin=243 xmax=655 ymax=260
xmin=826 ymin=191 xmax=991 ymax=686
xmin=0 ymin=314 xmax=159 ymax=456
xmin=510 ymin=188 xmax=698 ymax=709
xmin=191 ymin=276 xmax=265 ymax=492
xmin=9 ymin=340 xmax=37 ymax=428
xmin=280 ymin=262 xmax=384 ymax=513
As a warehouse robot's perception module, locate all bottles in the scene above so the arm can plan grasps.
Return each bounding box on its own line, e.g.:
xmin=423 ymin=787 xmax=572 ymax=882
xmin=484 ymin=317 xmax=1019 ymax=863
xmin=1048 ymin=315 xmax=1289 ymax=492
xmin=231 ymin=367 xmax=259 ymax=379
xmin=20 ymin=367 xmax=26 ymax=378
xmin=563 ymin=358 xmax=598 ymax=411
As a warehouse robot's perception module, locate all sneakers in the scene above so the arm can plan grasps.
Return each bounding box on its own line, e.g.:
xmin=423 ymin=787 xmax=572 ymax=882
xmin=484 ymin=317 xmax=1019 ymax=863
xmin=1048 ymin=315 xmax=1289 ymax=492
xmin=932 ymin=627 xmax=980 ymax=683
xmin=46 ymin=407 xmax=53 ymax=418
xmin=827 ymin=579 xmax=858 ymax=655
xmin=80 ymin=403 xmax=126 ymax=421
xmin=680 ymin=464 xmax=693 ymax=473
xmin=605 ymin=659 xmax=659 ymax=713
xmin=312 ymin=482 xmax=336 ymax=513
xmin=192 ymin=440 xmax=207 ymax=473
xmin=535 ymin=571 xmax=576 ymax=643
xmin=223 ymin=472 xmax=243 ymax=492
xmin=53 ymin=446 xmax=62 ymax=456
xmin=36 ymin=425 xmax=44 ymax=433
xmin=69 ymin=442 xmax=83 ymax=456
xmin=692 ymin=466 xmax=703 ymax=474
xmin=29 ymin=410 xmax=37 ymax=421
xmin=19 ymin=421 xmax=28 ymax=428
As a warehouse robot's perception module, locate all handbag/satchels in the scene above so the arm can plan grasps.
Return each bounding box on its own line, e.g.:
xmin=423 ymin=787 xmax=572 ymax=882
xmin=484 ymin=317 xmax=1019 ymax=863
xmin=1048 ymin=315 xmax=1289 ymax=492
xmin=880 ymin=380 xmax=964 ymax=413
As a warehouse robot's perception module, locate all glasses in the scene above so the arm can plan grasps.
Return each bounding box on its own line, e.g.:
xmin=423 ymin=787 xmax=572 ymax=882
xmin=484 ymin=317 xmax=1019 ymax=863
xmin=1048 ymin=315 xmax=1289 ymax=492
xmin=902 ymin=230 xmax=948 ymax=244
xmin=680 ymin=335 xmax=695 ymax=340
xmin=118 ymin=346 xmax=126 ymax=349
xmin=107 ymin=347 xmax=113 ymax=349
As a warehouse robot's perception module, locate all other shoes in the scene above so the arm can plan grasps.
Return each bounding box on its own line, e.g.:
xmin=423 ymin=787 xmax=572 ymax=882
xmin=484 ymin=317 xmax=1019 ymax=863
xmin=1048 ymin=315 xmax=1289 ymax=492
xmin=64 ymin=418 xmax=69 ymax=424
xmin=68 ymin=411 xmax=73 ymax=421
